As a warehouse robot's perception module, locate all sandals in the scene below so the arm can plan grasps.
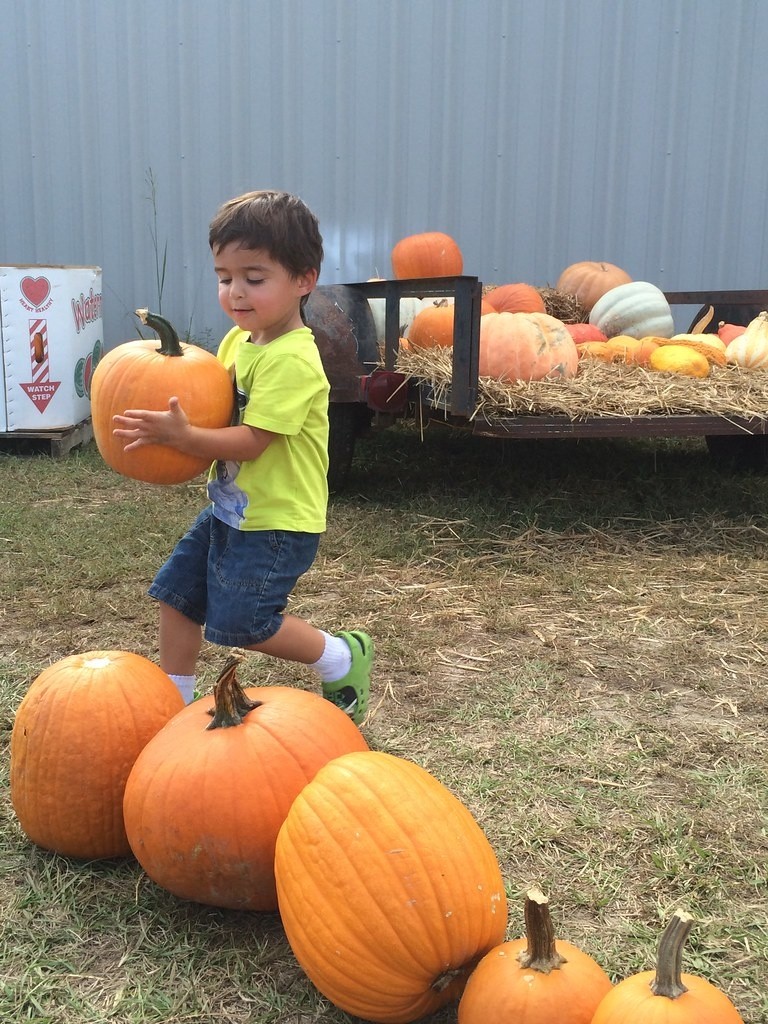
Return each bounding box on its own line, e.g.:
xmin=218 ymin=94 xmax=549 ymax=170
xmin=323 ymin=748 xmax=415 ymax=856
xmin=321 ymin=631 xmax=373 ymax=726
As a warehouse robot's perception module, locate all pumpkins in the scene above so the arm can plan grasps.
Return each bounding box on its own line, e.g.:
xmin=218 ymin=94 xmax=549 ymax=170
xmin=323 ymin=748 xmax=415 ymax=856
xmin=365 ymin=232 xmax=768 ymax=382
xmin=9 ymin=651 xmax=746 ymax=1024
xmin=89 ymin=309 xmax=234 ymax=484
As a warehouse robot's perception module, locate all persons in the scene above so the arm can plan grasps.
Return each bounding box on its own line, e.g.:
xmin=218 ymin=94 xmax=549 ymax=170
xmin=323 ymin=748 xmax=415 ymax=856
xmin=111 ymin=189 xmax=372 ymax=726
